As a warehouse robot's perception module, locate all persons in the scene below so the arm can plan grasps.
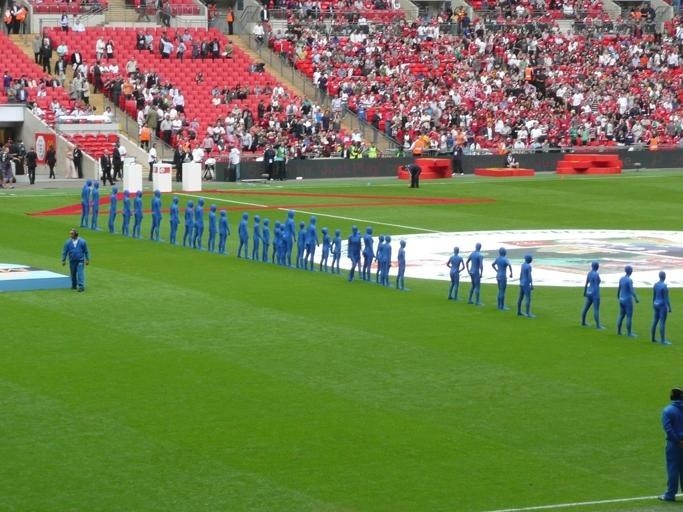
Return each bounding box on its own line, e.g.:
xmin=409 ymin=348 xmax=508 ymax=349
xmin=466 ymin=243 xmax=484 ymax=305
xmin=447 ymin=246 xmax=465 ymax=301
xmin=517 ymin=255 xmax=536 ymax=318
xmin=1 ymin=1 xmax=683 ymax=187
xmin=492 ymin=247 xmax=512 ymax=310
xmin=61 ymin=227 xmax=89 ymax=291
xmin=617 ymin=266 xmax=639 ymax=338
xmin=78 ymin=180 xmax=405 ymax=290
xmin=658 ymin=386 xmax=683 ymax=502
xmin=580 ymin=260 xmax=607 ymax=330
xmin=651 ymin=271 xmax=673 ymax=345
